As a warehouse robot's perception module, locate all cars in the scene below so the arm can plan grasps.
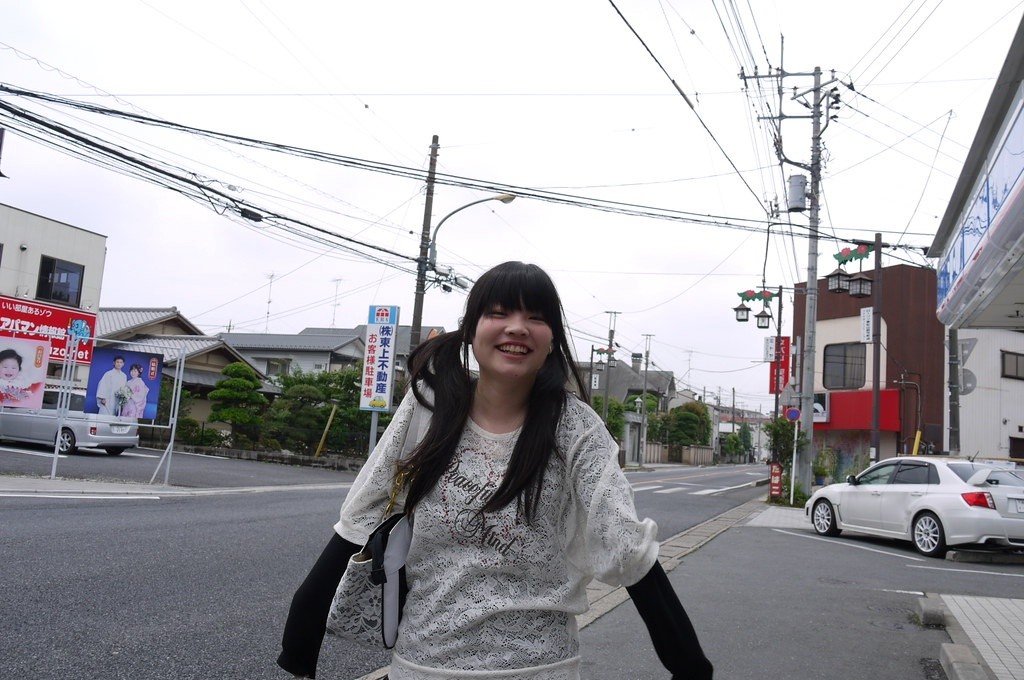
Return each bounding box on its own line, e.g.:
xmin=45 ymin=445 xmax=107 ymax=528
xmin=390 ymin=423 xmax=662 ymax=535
xmin=803 ymin=451 xmax=1023 ymax=557
xmin=0 ymin=389 xmax=139 ymax=456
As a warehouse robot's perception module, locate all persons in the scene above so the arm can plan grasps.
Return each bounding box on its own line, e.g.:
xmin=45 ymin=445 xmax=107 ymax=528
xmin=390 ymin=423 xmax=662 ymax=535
xmin=276 ymin=260 xmax=716 ymax=680
xmin=95 ymin=356 xmax=127 ymax=417
xmin=0 ymin=349 xmax=32 ymax=405
xmin=119 ymin=363 xmax=150 ymax=419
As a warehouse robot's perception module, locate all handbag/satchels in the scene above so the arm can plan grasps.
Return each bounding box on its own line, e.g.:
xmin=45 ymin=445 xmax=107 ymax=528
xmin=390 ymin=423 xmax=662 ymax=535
xmin=325 ymin=505 xmax=416 ymax=649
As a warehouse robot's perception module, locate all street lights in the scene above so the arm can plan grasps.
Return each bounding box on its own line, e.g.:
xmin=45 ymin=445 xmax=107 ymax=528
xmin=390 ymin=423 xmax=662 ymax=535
xmin=823 ymin=232 xmax=882 ymax=470
xmin=587 ymin=344 xmax=617 ymax=406
xmin=406 ymin=135 xmax=516 ymax=394
xmin=733 ymin=285 xmax=784 ymax=463
